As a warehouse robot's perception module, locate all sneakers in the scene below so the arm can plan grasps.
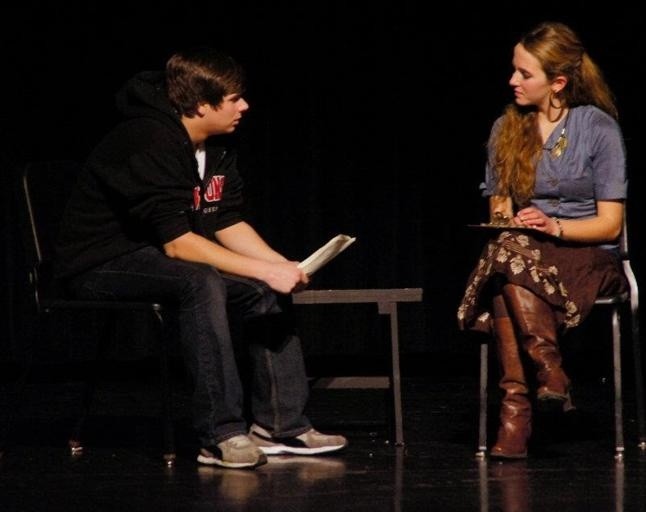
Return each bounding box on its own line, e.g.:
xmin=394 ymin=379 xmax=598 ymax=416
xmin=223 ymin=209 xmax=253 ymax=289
xmin=246 ymin=422 xmax=348 ymax=455
xmin=197 ymin=434 xmax=267 ymax=469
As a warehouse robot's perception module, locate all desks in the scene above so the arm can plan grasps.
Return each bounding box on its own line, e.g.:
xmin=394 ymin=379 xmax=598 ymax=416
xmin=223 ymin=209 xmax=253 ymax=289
xmin=290 ymin=287 xmax=423 ymax=446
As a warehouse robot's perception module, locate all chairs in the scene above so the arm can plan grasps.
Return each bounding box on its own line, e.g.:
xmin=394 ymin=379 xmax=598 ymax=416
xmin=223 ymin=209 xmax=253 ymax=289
xmin=23 ymin=165 xmax=178 ymax=465
xmin=474 ymin=180 xmax=639 ymax=453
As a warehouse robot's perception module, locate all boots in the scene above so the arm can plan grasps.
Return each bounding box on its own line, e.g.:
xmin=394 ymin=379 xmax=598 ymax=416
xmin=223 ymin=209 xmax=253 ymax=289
xmin=489 ymin=317 xmax=534 ymax=459
xmin=503 ymin=283 xmax=577 ymax=412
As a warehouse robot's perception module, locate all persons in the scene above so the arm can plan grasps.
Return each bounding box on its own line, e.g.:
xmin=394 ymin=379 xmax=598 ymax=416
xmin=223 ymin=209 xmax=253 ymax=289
xmin=38 ymin=41 xmax=349 ymax=468
xmin=457 ymin=22 xmax=629 ymax=459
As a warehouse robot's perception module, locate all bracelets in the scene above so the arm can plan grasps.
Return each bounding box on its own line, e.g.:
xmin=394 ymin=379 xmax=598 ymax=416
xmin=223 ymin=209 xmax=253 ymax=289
xmin=553 ymin=216 xmax=565 ymax=239
